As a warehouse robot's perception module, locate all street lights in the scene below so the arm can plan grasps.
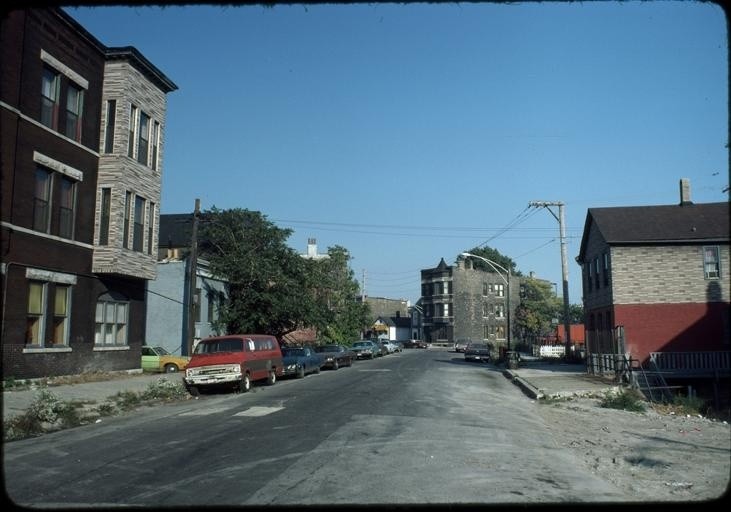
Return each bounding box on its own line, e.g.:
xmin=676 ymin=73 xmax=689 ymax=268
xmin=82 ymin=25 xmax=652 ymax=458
xmin=398 ymin=301 xmax=423 ymax=340
xmin=460 ymin=252 xmax=513 ymax=366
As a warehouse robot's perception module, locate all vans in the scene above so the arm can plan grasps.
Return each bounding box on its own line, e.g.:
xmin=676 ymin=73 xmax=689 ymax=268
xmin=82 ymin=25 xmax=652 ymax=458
xmin=185 ymin=334 xmax=284 ymax=397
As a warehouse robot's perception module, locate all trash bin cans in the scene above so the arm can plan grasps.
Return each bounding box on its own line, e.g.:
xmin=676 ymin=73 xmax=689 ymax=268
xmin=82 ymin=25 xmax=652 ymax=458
xmin=506 ymin=351 xmax=519 ymax=369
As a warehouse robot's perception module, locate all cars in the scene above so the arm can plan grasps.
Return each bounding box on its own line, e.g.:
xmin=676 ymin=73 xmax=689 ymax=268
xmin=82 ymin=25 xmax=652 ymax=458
xmin=351 ymin=341 xmax=379 ymax=360
xmin=405 ymin=338 xmax=432 ymax=349
xmin=277 ymin=344 xmax=326 ymax=380
xmin=341 ymin=345 xmax=357 ymax=363
xmin=464 ymin=342 xmax=492 ymax=363
xmin=376 ymin=340 xmax=405 ymax=357
xmin=455 ymin=339 xmax=472 ymax=353
xmin=314 ymin=345 xmax=358 ymax=370
xmin=141 ymin=344 xmax=194 ymax=374
